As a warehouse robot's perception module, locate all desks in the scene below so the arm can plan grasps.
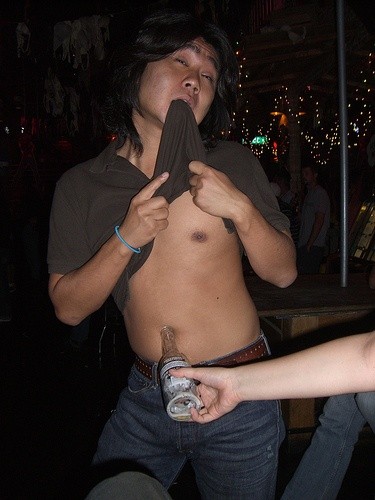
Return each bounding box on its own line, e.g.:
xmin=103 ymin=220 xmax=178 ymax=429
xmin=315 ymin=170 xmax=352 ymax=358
xmin=242 ymin=268 xmax=375 ymax=460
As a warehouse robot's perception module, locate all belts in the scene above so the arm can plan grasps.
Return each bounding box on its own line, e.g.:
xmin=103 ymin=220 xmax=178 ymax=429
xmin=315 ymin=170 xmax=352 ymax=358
xmin=134 ymin=338 xmax=267 ymax=384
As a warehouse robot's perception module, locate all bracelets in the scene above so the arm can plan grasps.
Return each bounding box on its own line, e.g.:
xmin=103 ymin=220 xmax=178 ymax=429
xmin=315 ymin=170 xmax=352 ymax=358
xmin=115 ymin=226 xmax=141 ymax=254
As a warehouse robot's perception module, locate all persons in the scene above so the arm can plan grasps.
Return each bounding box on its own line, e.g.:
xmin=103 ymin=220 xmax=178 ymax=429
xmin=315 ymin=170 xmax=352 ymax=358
xmin=24 ymin=216 xmax=41 ymax=279
xmin=170 ymin=330 xmax=375 ymax=500
xmin=48 ymin=12 xmax=298 ymax=499
xmin=274 ymin=166 xmax=331 ymax=272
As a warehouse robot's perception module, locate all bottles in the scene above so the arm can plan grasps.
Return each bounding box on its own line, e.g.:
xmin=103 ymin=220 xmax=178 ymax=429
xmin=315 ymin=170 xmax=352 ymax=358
xmin=157 ymin=328 xmax=201 ymax=421
